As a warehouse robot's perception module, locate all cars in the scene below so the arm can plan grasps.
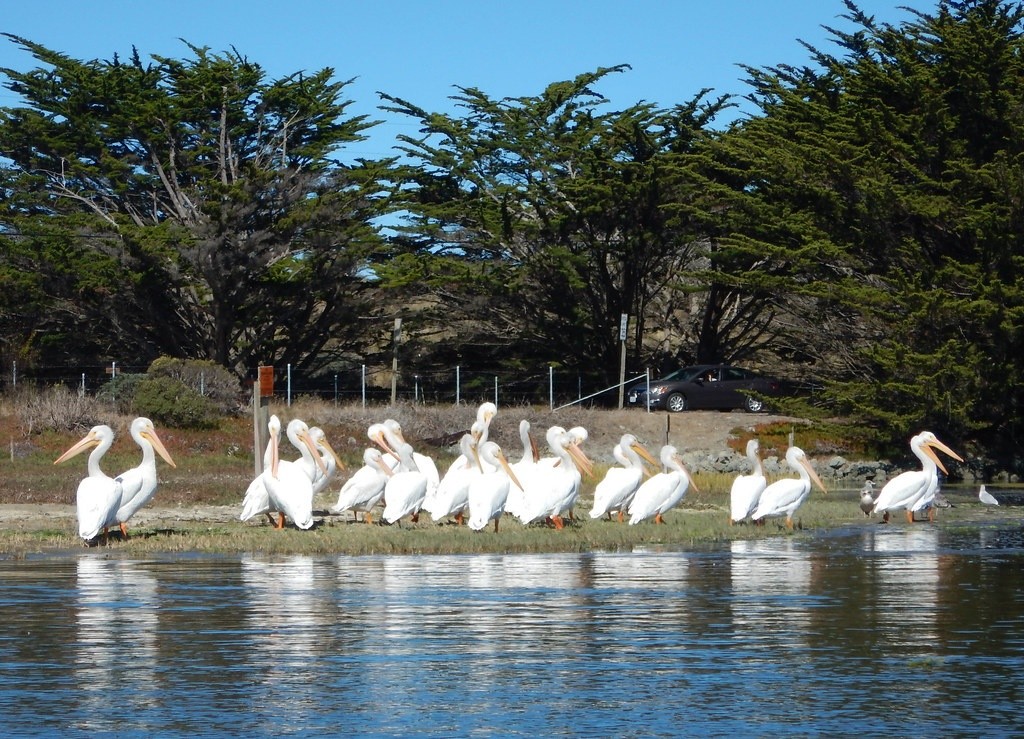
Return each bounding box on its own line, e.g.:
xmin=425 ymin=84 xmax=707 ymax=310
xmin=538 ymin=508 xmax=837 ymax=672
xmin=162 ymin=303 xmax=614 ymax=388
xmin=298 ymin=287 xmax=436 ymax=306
xmin=628 ymin=365 xmax=779 ymax=413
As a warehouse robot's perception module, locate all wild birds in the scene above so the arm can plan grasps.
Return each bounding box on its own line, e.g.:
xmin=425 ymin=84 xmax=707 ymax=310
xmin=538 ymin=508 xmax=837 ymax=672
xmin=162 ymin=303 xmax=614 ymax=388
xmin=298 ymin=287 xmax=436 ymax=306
xmin=728 ymin=440 xmax=828 ymax=530
xmin=53 ymin=417 xmax=176 ymax=545
xmin=873 ymin=431 xmax=965 ymax=523
xmin=859 ymin=480 xmax=876 ymax=518
xmin=588 ymin=433 xmax=699 ymax=527
xmin=978 ymin=484 xmax=1000 ymax=511
xmin=240 ymin=415 xmax=345 ymax=532
xmin=328 ymin=402 xmax=595 ymax=534
